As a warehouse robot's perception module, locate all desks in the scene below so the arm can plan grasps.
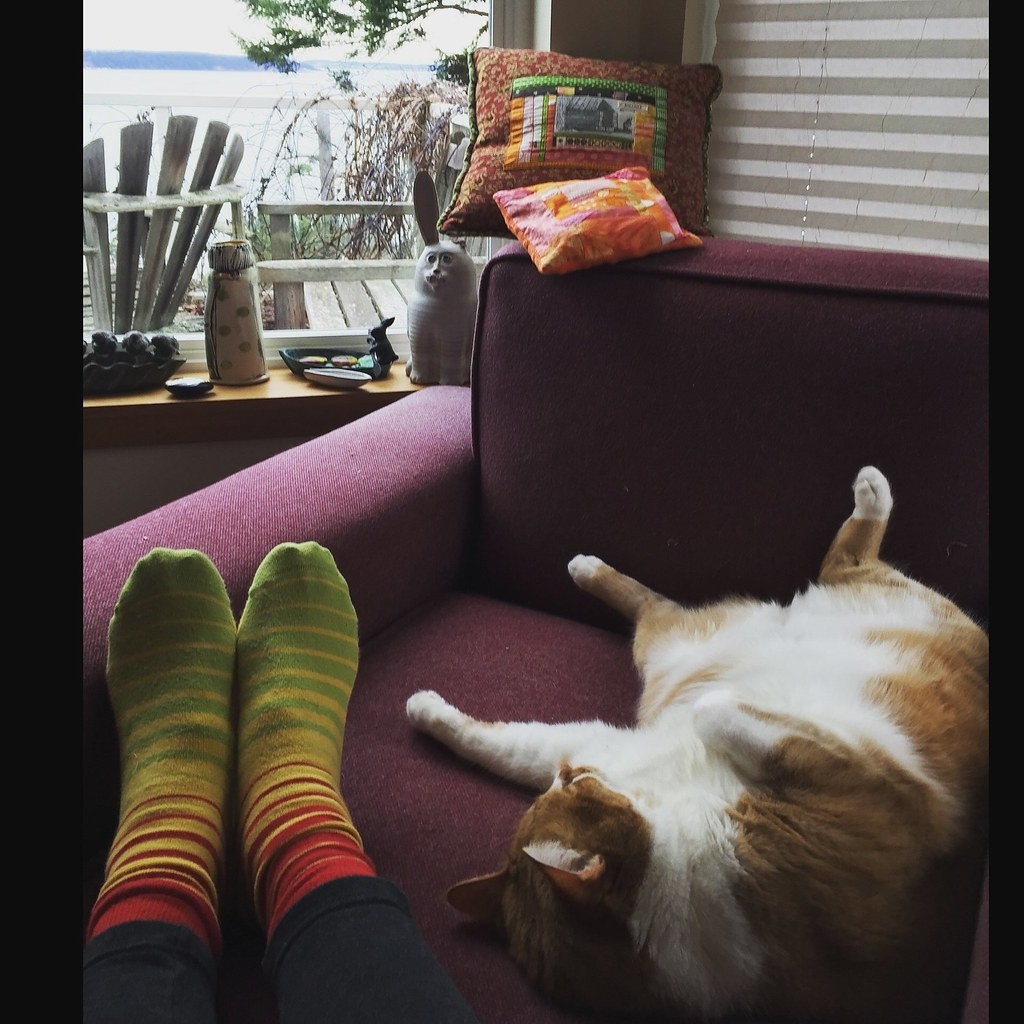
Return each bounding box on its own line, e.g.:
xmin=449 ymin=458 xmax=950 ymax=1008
xmin=82 ymin=362 xmax=426 ymax=537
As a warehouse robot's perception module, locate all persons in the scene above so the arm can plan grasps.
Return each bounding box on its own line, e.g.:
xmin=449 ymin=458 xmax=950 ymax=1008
xmin=82 ymin=540 xmax=481 ymax=1022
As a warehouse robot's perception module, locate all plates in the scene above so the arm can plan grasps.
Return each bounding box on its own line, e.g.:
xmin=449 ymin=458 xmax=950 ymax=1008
xmin=304 ymin=368 xmax=373 ymax=389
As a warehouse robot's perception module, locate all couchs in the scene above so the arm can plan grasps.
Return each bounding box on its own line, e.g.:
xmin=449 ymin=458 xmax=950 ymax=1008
xmin=80 ymin=238 xmax=989 ymax=1024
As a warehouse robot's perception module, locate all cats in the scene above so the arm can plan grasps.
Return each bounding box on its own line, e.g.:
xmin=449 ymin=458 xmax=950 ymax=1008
xmin=408 ymin=464 xmax=993 ymax=1021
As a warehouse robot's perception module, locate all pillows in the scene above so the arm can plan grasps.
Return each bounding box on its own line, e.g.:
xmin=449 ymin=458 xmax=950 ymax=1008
xmin=436 ymin=47 xmax=724 ymax=238
xmin=496 ymin=167 xmax=700 ymax=273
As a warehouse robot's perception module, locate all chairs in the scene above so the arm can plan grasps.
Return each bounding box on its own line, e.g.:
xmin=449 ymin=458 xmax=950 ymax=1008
xmin=255 ymin=117 xmax=504 ymax=332
